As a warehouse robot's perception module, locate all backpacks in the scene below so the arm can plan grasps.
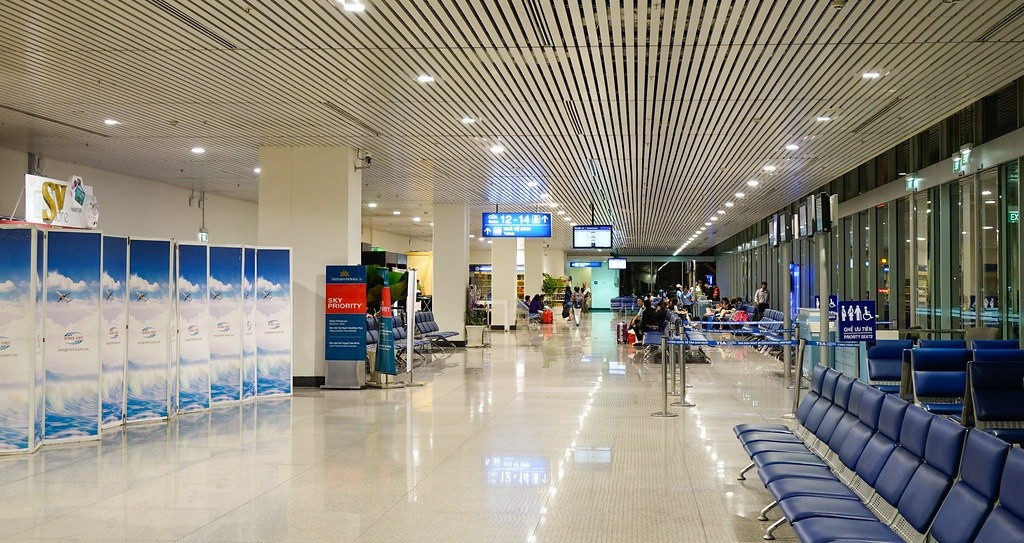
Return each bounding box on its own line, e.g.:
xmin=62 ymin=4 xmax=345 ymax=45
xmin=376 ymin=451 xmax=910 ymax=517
xmin=573 ymin=292 xmax=582 ymax=308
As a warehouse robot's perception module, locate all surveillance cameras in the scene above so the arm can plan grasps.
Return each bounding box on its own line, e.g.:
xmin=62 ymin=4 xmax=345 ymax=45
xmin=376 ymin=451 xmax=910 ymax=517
xmin=365 ymin=153 xmax=371 ymax=164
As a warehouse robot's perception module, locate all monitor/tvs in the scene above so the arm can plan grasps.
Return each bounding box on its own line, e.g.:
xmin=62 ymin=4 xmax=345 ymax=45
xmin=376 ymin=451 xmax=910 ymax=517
xmin=573 ymin=225 xmax=613 ymax=249
xmin=608 ymin=258 xmax=627 ymax=269
xmin=767 ymin=191 xmax=838 ymax=248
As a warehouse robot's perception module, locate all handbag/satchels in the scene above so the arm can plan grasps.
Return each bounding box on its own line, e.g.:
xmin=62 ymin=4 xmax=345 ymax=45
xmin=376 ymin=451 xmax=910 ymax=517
xmin=628 ymin=329 xmax=635 ymax=344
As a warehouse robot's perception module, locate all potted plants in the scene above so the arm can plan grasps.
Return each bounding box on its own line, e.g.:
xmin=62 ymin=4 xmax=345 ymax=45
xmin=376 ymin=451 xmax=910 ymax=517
xmin=465 ymin=310 xmax=487 ymax=347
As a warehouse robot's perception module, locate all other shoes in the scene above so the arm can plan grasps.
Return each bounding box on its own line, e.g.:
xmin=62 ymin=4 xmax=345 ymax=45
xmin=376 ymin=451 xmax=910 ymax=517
xmin=576 ymin=323 xmax=579 ymax=326
xmin=633 ymin=342 xmax=643 ymax=346
xmin=562 ymin=308 xmax=570 ymax=318
xmin=567 ymin=317 xmax=572 ymax=321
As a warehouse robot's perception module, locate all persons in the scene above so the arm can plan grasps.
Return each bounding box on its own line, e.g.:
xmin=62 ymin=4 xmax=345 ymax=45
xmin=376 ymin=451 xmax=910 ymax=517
xmin=528 ymin=294 xmax=544 ymax=318
xmin=703 ymin=283 xmax=712 ymax=300
xmin=680 ymin=286 xmax=695 ymax=317
xmin=562 ymin=286 xmax=573 ymax=323
xmin=712 ymin=283 xmax=720 ymax=302
xmin=754 ymin=282 xmax=770 ymax=320
xmin=731 ymin=299 xmax=736 ymax=310
xmin=644 ymin=294 xmax=656 ymax=301
xmin=570 ymin=286 xmax=584 ymax=326
xmin=676 ymin=284 xmax=684 ymax=309
xmin=523 ymin=295 xmax=532 ymax=306
xmin=718 ymin=297 xmax=731 ymax=330
xmin=632 ymin=300 xmax=661 ymax=346
xmin=581 ymin=282 xmax=592 ymax=314
xmin=736 ymin=297 xmax=743 ymax=311
xmin=627 ymin=297 xmax=646 ymax=331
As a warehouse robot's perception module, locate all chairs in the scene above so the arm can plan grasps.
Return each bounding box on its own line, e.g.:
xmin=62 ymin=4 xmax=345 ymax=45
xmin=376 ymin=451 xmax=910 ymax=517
xmin=866 ymin=336 xmax=1024 ymax=447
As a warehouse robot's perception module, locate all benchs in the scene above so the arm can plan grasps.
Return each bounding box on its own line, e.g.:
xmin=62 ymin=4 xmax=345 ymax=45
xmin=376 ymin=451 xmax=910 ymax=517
xmin=732 ymin=362 xmax=1023 ymax=543
xmin=611 ymin=296 xmax=711 ymax=362
xmin=729 ymin=304 xmax=798 ymax=360
xmin=518 ymin=303 xmax=540 ymax=329
xmin=366 ymin=312 xmax=459 ymax=371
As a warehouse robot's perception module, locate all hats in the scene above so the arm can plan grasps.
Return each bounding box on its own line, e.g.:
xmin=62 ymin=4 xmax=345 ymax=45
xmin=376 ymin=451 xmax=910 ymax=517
xmin=676 ymin=284 xmax=682 ymax=288
xmin=634 ymin=296 xmax=644 ymax=303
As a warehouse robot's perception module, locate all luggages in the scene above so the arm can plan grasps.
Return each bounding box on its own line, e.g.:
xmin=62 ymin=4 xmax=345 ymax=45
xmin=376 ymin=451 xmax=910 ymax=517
xmin=617 ymin=320 xmax=628 ymax=344
xmin=543 ymin=309 xmax=553 ymax=324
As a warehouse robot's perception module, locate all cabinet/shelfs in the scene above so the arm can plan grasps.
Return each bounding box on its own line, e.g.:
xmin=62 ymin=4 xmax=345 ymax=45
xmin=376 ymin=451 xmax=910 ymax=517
xmin=545 ymin=279 xmax=569 ymax=306
xmin=474 ymin=272 xmax=524 ymax=301
